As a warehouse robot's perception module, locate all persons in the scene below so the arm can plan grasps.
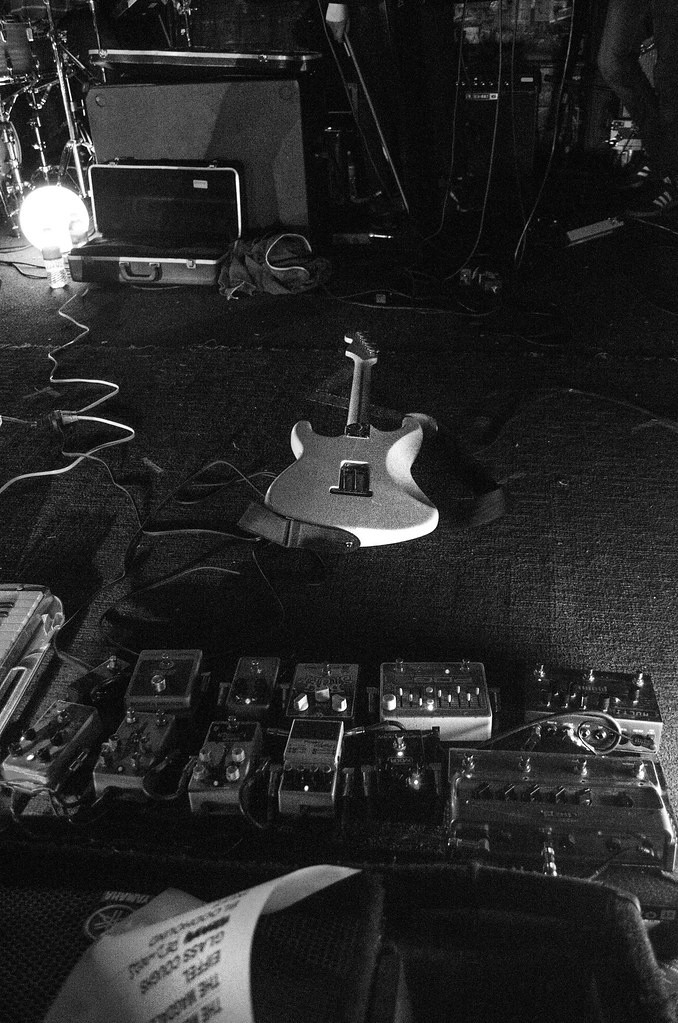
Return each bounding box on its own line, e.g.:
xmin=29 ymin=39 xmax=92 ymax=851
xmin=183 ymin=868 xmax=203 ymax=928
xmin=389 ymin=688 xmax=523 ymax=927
xmin=596 ymin=0 xmax=678 ymax=218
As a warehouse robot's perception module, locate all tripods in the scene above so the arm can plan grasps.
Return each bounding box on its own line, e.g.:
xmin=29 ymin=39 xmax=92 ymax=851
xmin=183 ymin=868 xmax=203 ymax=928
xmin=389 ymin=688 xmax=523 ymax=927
xmin=0 ymin=0 xmax=96 ymax=240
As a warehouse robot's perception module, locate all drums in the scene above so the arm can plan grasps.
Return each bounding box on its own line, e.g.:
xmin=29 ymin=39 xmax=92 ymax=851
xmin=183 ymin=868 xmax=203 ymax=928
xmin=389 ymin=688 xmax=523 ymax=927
xmin=1 ymin=14 xmax=62 ymax=84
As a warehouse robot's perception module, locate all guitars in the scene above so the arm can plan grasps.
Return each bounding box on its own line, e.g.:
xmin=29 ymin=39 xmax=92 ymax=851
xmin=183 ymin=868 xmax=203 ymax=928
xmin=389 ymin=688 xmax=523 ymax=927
xmin=264 ymin=327 xmax=444 ymax=545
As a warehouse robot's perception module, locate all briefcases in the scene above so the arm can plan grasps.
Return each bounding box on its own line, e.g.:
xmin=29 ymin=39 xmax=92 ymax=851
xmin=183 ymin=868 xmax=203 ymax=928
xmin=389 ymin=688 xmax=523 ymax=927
xmin=67 ymin=157 xmax=242 ymax=286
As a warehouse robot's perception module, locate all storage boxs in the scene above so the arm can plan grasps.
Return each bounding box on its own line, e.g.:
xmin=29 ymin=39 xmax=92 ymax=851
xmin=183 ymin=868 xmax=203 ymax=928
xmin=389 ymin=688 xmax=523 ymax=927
xmin=346 ymin=864 xmax=677 ymax=1023
